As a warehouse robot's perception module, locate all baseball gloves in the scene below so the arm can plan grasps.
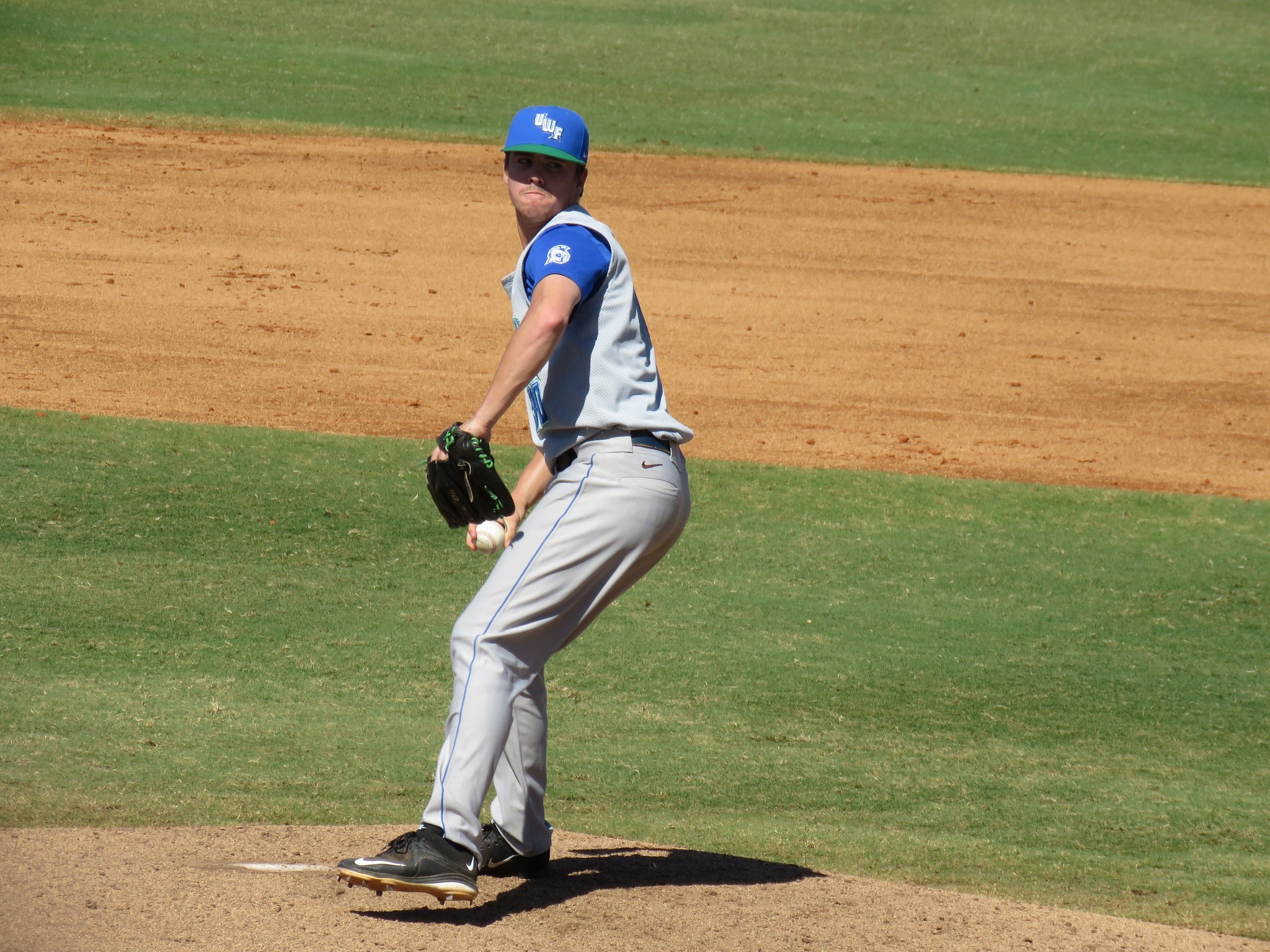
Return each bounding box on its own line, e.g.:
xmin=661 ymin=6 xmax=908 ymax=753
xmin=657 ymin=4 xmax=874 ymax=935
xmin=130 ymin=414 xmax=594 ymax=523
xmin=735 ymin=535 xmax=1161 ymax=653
xmin=426 ymin=419 xmax=517 ymax=529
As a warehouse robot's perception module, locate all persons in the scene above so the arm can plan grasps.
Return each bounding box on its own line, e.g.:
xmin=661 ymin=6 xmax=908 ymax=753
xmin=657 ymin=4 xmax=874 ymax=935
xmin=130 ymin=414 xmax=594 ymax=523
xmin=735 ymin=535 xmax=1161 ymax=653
xmin=336 ymin=105 xmax=695 ymax=905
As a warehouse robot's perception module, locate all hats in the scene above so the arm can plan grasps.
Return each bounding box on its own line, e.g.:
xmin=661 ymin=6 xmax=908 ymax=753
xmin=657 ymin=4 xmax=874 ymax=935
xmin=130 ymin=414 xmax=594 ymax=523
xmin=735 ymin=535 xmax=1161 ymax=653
xmin=501 ymin=106 xmax=589 ymax=166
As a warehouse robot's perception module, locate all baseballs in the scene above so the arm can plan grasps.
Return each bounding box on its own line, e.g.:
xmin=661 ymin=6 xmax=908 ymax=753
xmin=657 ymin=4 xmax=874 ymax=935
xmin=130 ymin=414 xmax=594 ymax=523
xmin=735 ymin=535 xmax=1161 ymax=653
xmin=471 ymin=519 xmax=505 ymax=551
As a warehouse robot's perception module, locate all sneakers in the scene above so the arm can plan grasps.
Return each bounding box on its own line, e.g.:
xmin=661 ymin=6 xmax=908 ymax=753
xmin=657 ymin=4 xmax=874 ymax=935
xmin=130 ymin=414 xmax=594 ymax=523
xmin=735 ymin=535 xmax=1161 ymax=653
xmin=477 ymin=819 xmax=550 ymax=879
xmin=336 ymin=823 xmax=478 ymax=906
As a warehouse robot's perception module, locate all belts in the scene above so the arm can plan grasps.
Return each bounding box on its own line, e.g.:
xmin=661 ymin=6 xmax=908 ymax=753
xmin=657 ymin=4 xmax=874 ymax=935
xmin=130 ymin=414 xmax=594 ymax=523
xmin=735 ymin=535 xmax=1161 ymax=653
xmin=552 ymin=430 xmax=670 ymax=475
